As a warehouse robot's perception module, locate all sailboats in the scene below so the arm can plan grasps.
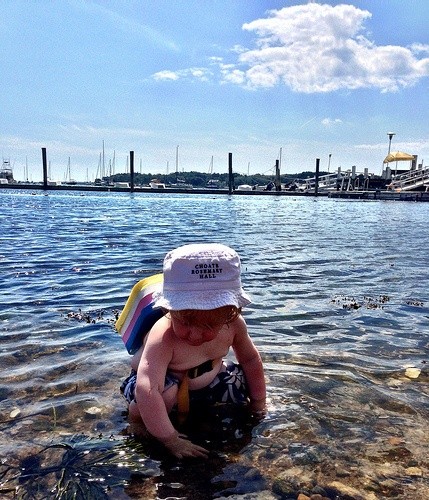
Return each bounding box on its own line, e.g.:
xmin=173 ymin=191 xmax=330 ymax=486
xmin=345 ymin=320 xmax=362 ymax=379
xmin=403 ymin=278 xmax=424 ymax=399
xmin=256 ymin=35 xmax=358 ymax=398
xmin=60 ymin=154 xmax=77 ymax=185
xmin=205 ymin=155 xmax=222 ymax=189
xmin=84 ymin=140 xmax=151 ymax=187
xmin=237 ymin=161 xmax=253 ymax=191
xmin=0 ymin=154 xmax=33 ymax=184
xmin=46 ymin=160 xmax=56 ymax=186
xmin=169 ymin=144 xmax=193 ymax=188
xmin=254 ymin=147 xmax=290 ymax=190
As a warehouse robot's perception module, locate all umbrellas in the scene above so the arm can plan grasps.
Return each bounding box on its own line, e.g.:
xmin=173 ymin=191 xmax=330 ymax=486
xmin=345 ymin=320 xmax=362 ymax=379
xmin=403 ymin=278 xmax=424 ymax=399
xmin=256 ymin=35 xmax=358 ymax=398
xmin=383 ymin=150 xmax=415 ymax=183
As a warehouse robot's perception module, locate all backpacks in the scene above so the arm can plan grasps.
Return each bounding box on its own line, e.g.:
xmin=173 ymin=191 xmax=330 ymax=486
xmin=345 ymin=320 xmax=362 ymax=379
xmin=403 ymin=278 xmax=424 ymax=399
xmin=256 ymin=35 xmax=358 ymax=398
xmin=115 ymin=274 xmax=169 ymax=355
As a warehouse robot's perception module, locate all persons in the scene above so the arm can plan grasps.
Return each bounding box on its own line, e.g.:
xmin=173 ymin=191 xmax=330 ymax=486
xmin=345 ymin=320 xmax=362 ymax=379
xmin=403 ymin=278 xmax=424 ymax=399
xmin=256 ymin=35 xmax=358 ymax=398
xmin=120 ymin=246 xmax=265 ymax=457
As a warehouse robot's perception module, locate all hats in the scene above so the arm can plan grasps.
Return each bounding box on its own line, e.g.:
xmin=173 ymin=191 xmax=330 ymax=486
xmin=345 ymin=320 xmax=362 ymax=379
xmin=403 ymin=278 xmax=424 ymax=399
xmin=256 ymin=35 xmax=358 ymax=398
xmin=152 ymin=244 xmax=251 ymax=310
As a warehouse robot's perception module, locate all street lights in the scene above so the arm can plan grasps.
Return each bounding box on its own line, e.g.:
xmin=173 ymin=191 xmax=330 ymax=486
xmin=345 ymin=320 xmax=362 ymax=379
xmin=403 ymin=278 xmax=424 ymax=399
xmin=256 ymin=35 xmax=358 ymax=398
xmin=385 ymin=132 xmax=397 ymax=181
